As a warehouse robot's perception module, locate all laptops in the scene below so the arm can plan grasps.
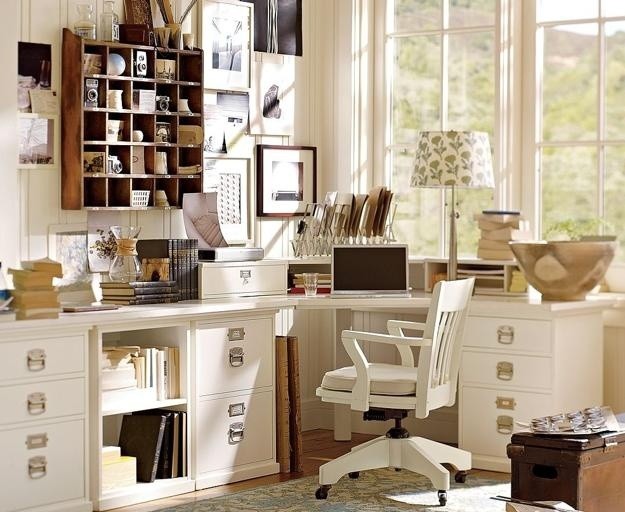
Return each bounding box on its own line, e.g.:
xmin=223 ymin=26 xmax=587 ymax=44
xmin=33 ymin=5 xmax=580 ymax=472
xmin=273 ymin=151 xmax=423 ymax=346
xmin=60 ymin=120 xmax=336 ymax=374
xmin=329 ymin=243 xmax=411 ymax=299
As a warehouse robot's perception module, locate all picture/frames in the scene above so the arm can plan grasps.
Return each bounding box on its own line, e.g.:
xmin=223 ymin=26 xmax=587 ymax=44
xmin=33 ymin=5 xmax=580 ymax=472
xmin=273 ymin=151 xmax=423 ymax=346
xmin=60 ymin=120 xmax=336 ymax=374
xmin=256 ymin=145 xmax=317 ymax=217
xmin=203 ymin=155 xmax=254 ymax=245
xmin=196 ymin=0 xmax=254 ymax=96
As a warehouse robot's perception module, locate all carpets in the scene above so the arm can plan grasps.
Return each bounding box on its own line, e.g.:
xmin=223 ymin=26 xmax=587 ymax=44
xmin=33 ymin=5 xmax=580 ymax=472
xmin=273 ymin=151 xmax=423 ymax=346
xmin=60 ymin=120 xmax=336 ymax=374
xmin=152 ymin=468 xmax=511 ymax=512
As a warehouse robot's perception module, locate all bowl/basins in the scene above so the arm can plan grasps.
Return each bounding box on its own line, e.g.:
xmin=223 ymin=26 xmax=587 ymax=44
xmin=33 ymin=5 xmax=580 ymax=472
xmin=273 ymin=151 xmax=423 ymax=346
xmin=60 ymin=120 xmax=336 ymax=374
xmin=508 ymin=239 xmax=619 ymax=301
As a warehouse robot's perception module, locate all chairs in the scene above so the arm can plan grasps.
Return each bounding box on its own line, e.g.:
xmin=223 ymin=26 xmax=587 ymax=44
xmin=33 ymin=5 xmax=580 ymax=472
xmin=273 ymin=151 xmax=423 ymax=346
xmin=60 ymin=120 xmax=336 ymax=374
xmin=314 ymin=277 xmax=478 ymax=505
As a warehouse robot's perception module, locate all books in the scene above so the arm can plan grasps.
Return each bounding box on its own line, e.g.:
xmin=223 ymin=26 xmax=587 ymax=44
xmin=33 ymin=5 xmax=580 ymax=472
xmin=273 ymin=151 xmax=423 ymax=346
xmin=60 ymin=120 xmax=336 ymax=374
xmin=62 ymin=280 xmax=181 ymax=314
xmin=136 ymin=238 xmax=199 ymax=301
xmin=6 ymin=256 xmax=64 ymax=322
xmin=101 ymin=340 xmax=180 ymax=413
xmin=99 ymin=408 xmax=188 ymax=493
xmin=473 ymin=207 xmax=534 ymax=261
xmin=288 ymin=272 xmax=332 ymax=293
xmin=456 ymin=268 xmax=505 ymax=295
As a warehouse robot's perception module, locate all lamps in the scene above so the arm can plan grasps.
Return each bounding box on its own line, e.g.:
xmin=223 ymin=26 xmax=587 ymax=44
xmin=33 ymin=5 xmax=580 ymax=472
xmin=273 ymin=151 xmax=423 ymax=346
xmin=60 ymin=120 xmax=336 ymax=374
xmin=410 ymin=131 xmax=495 ymax=281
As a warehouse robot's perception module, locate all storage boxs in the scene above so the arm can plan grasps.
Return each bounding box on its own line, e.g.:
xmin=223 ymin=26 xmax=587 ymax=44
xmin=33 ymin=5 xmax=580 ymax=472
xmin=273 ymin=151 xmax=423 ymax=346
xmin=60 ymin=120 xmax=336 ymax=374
xmin=507 ymin=419 xmax=625 ymax=512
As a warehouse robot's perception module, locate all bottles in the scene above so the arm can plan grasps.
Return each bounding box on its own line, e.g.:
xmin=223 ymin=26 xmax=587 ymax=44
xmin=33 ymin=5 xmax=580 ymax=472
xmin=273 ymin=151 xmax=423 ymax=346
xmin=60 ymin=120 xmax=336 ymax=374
xmin=74 ymin=3 xmax=97 ymax=42
xmin=100 ymin=1 xmax=120 ymax=42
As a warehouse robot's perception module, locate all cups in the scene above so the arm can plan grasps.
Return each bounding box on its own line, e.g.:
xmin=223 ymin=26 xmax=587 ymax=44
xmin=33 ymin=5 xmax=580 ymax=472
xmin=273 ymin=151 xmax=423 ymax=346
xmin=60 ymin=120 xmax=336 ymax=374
xmin=166 ymin=23 xmax=181 ymax=49
xmin=303 ymin=273 xmax=318 ymax=296
xmin=153 ymin=28 xmax=170 ymax=47
xmin=183 ymin=34 xmax=193 ymax=51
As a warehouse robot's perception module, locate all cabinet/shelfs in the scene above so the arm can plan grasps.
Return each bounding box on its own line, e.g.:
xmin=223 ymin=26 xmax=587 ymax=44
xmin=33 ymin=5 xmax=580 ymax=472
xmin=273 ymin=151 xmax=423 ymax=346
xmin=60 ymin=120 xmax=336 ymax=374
xmin=1 ymin=314 xmax=93 ymax=512
xmin=296 ymin=298 xmax=617 ymax=473
xmin=193 ymin=304 xmax=280 ymax=490
xmin=91 ymin=312 xmax=196 ymax=509
xmin=61 ymin=27 xmax=205 ymax=213
